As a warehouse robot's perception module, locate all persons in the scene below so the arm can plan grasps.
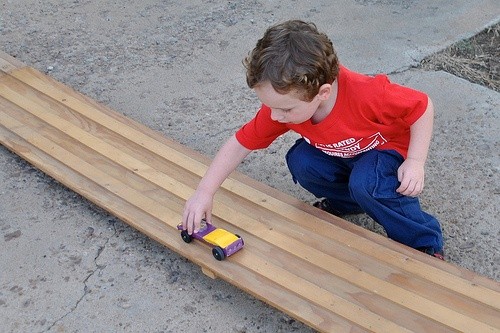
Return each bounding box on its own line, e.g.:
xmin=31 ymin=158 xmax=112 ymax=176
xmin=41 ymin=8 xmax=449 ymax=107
xmin=180 ymin=20 xmax=445 ymax=261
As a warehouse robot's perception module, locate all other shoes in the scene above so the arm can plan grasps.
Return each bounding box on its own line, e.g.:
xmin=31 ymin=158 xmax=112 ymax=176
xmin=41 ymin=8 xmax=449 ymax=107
xmin=314 ymin=199 xmax=366 ymax=216
